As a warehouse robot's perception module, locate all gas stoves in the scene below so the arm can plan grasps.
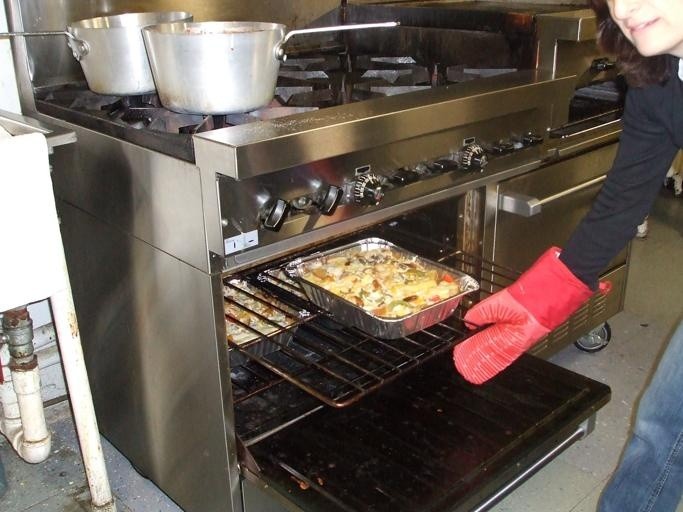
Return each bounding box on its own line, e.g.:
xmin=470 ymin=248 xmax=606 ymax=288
xmin=33 ymin=39 xmax=523 ymax=133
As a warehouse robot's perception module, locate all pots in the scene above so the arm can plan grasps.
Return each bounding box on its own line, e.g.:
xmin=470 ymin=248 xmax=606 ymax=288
xmin=140 ymin=20 xmax=402 ymax=114
xmin=1 ymin=10 xmax=195 ymax=95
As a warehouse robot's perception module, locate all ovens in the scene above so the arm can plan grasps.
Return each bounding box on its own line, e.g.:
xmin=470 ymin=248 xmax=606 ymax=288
xmin=223 ymin=189 xmax=612 ymax=512
xmin=479 ymin=135 xmax=631 ymax=360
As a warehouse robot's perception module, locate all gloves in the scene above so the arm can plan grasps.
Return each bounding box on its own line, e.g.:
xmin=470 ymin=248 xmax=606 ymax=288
xmin=452 ymin=245 xmax=592 ymax=385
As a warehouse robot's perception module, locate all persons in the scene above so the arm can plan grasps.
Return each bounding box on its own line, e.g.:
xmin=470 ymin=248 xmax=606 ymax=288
xmin=454 ymin=0 xmax=683 ymax=512
